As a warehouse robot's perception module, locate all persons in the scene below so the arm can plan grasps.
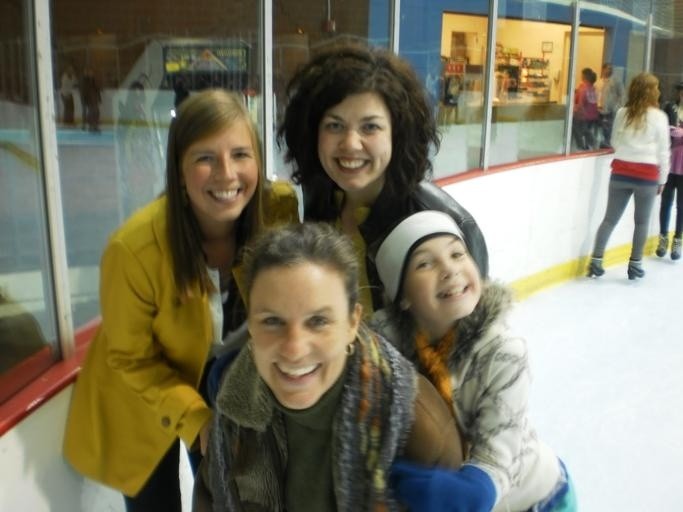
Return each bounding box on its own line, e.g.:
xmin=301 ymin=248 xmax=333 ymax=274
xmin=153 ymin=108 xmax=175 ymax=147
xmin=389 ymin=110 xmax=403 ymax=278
xmin=63 ymin=61 xmax=104 ymax=134
xmin=190 ymin=223 xmax=462 ymax=511
xmin=575 ymin=64 xmax=682 ymax=278
xmin=63 ymin=90 xmax=299 ymax=511
xmin=364 ymin=207 xmax=578 ymax=511
xmin=172 ymin=77 xmax=190 ymax=116
xmin=273 ymin=45 xmax=489 ymax=316
xmin=442 ymin=73 xmax=465 ymax=125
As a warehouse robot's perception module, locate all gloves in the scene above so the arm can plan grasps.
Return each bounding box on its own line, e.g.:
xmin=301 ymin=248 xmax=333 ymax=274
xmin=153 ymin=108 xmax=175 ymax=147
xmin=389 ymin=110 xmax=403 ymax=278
xmin=388 ymin=466 xmax=496 ymax=511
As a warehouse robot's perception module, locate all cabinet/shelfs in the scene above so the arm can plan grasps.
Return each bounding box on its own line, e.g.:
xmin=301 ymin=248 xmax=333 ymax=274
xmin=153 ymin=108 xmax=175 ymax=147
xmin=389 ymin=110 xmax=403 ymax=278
xmin=492 ymin=42 xmax=522 ymax=95
xmin=519 ymin=56 xmax=550 ymax=98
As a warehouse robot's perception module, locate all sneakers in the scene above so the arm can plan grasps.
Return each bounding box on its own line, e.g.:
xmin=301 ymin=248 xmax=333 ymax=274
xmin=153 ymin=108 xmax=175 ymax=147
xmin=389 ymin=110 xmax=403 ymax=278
xmin=627 ymin=259 xmax=644 ymax=279
xmin=656 ymin=234 xmax=681 ymax=259
xmin=588 ymin=257 xmax=603 ymax=277
